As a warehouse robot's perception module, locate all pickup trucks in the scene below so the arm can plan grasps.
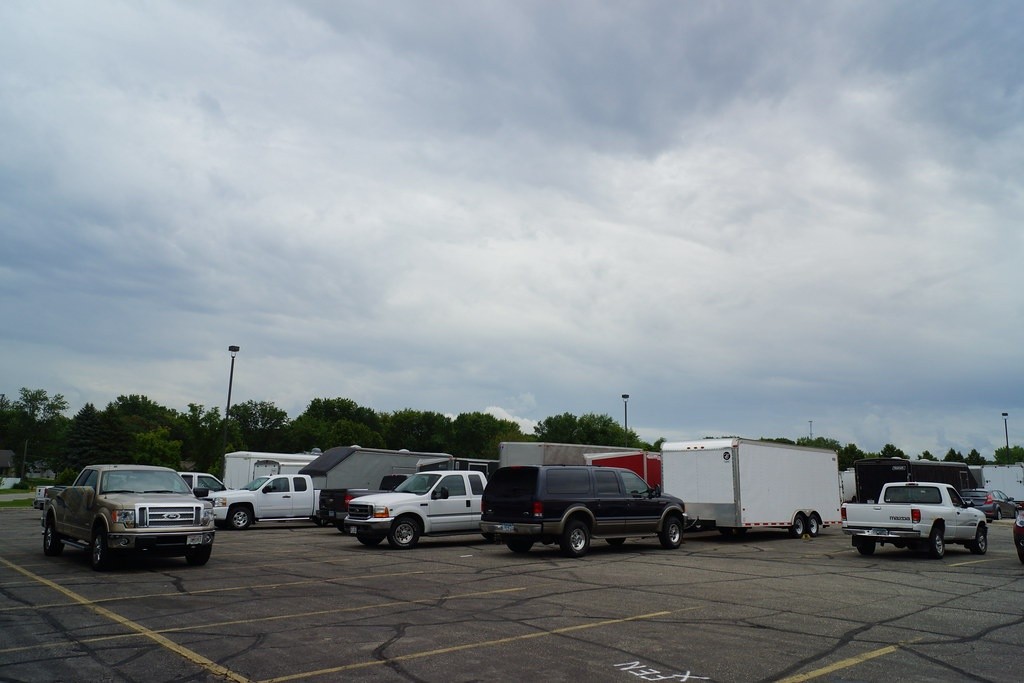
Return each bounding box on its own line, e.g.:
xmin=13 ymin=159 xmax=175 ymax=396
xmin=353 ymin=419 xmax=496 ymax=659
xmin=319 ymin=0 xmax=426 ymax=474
xmin=319 ymin=474 xmax=415 ymax=535
xmin=203 ymin=474 xmax=322 ymax=531
xmin=840 ymin=482 xmax=988 ymax=560
xmin=478 ymin=464 xmax=688 ymax=559
xmin=138 ymin=472 xmax=230 ymax=498
xmin=30 ymin=474 xmax=138 ymax=510
xmin=343 ymin=470 xmax=505 ymax=550
xmin=40 ymin=464 xmax=215 ymax=572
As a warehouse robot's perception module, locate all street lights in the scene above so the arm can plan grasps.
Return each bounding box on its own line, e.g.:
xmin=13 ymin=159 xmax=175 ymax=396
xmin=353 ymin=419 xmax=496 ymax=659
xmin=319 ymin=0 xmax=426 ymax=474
xmin=809 ymin=420 xmax=815 ymax=441
xmin=1001 ymin=412 xmax=1010 ymax=464
xmin=622 ymin=394 xmax=630 ymax=447
xmin=223 ymin=346 xmax=240 ymax=450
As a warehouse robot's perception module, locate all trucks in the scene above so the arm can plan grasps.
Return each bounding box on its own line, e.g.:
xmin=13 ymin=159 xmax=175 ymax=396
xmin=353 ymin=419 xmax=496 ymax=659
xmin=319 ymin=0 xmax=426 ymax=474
xmin=301 ymin=444 xmax=501 ymax=492
xmin=660 ymin=437 xmax=844 ymax=540
xmin=498 ymin=440 xmax=660 ymax=467
xmin=852 ymin=458 xmax=978 ymax=504
xmin=224 ymin=448 xmax=328 ymax=526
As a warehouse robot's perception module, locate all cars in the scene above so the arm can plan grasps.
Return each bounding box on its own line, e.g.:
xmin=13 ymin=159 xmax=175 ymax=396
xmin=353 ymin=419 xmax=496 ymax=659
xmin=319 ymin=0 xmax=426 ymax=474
xmin=959 ymin=489 xmax=1019 ymax=521
